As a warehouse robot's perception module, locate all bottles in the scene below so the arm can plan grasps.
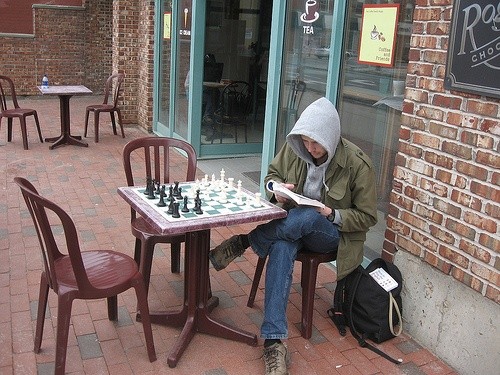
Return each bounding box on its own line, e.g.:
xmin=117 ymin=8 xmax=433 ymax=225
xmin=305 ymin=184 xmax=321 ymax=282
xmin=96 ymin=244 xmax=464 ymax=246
xmin=41 ymin=74 xmax=48 ymax=92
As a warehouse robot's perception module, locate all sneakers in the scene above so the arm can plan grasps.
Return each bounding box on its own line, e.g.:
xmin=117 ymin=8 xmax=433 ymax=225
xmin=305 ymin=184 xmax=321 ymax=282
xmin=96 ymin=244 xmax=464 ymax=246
xmin=208 ymin=234 xmax=245 ymax=271
xmin=262 ymin=339 xmax=288 ymax=375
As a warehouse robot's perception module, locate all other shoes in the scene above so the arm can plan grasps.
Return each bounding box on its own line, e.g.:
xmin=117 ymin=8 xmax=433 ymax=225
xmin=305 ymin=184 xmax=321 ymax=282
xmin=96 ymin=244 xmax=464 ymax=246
xmin=202 ymin=112 xmax=214 ymax=125
xmin=214 ymin=112 xmax=226 ymax=121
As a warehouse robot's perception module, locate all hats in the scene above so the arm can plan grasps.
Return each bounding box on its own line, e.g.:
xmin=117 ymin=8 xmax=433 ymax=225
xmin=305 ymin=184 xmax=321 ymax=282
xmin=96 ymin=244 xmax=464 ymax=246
xmin=204 ymin=54 xmax=216 ymax=65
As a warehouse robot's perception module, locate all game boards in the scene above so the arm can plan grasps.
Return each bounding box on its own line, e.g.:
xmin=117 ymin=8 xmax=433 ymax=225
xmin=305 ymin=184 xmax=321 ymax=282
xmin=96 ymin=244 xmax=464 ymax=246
xmin=131 ymin=180 xmax=273 ymax=223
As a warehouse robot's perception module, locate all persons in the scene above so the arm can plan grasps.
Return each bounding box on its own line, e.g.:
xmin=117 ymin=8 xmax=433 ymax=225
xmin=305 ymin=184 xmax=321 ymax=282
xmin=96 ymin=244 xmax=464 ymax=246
xmin=182 ymin=54 xmax=221 ymax=124
xmin=209 ymin=98 xmax=376 ymax=375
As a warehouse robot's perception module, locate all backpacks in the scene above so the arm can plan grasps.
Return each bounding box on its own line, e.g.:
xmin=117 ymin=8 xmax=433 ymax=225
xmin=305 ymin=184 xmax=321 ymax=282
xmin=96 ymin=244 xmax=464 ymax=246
xmin=327 ymin=258 xmax=403 ymax=346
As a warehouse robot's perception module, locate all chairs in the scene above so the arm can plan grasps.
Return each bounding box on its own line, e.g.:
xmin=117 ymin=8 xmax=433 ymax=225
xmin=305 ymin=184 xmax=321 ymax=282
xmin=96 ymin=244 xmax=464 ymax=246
xmin=14 ymin=176 xmax=161 ymax=375
xmin=123 ymin=137 xmax=198 ymax=310
xmin=277 ymin=80 xmax=306 ymax=139
xmin=0 ymin=76 xmax=44 ymax=151
xmin=211 ymin=80 xmax=251 ymax=143
xmin=85 ymin=73 xmax=126 ymax=143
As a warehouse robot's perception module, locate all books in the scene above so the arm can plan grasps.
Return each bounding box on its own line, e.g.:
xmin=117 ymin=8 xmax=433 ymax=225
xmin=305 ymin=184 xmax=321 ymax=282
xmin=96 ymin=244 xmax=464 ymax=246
xmin=266 ymin=179 xmax=325 ymax=209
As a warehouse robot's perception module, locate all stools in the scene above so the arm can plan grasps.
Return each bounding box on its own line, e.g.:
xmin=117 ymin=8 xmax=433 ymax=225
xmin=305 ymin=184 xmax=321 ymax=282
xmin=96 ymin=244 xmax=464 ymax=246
xmin=246 ymin=248 xmax=340 ymax=339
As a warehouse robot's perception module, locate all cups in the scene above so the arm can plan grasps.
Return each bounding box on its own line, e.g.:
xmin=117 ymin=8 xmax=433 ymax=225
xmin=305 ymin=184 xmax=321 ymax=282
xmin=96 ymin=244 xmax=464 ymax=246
xmin=393 ymin=80 xmax=405 ymax=97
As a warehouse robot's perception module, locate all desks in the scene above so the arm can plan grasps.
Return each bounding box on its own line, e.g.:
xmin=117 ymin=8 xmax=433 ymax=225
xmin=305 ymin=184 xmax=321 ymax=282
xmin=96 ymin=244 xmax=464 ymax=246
xmin=204 ymin=82 xmax=238 ymax=115
xmin=116 ymin=182 xmax=290 ymax=369
xmin=35 ymin=85 xmax=92 ymax=149
